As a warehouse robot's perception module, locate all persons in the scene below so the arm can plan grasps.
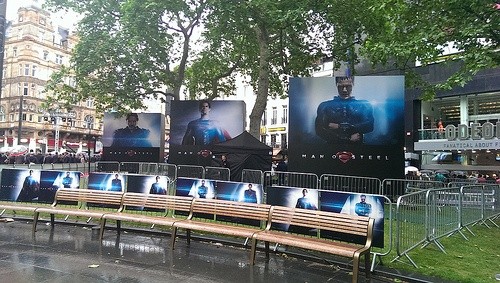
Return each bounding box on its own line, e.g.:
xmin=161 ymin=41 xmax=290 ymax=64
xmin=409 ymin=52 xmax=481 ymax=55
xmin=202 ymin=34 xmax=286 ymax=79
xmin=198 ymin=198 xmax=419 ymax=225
xmin=438 ymin=122 xmax=444 ymax=138
xmin=111 ymin=174 xmax=122 ymax=193
xmin=219 ymin=154 xmax=230 ymax=169
xmin=62 ymin=172 xmax=72 ymax=187
xmin=315 ymin=77 xmax=375 ymax=171
xmin=198 ymin=180 xmax=209 ymax=198
xmin=0 ymin=148 xmax=101 ymax=163
xmin=181 ymin=101 xmax=233 ymax=166
xmin=243 ymin=184 xmax=258 ymax=204
xmin=355 ymin=194 xmax=372 ymax=218
xmin=272 ymin=157 xmax=288 ymax=185
xmin=108 ymin=114 xmax=154 ymax=161
xmin=288 ymin=188 xmax=318 ymax=236
xmin=16 ymin=170 xmax=40 ymax=202
xmin=407 ymin=167 xmax=500 ymax=184
xmin=144 ymin=176 xmax=168 ymax=213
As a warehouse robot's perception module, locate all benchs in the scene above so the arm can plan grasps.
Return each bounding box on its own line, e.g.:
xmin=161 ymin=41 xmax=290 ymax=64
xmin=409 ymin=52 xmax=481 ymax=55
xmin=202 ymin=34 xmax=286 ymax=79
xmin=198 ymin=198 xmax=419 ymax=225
xmin=0 ymin=187 xmax=375 ymax=283
